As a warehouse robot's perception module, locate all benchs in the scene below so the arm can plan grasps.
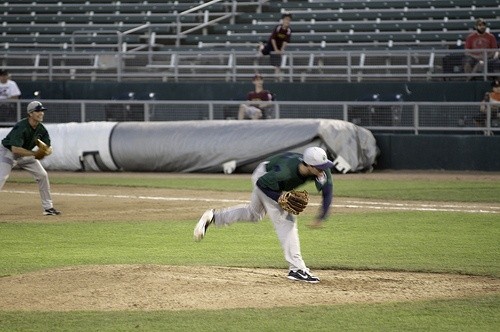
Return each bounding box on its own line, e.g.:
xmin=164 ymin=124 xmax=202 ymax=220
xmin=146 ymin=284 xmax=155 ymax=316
xmin=1 ymin=0 xmax=500 ymax=71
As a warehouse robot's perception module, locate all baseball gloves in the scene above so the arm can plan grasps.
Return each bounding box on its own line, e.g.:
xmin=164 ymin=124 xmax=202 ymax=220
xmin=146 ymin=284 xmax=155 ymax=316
xmin=278 ymin=189 xmax=309 ymax=215
xmin=35 ymin=138 xmax=49 ymax=160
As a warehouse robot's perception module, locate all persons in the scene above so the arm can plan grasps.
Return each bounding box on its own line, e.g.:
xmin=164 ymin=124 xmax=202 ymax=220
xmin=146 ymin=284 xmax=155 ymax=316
xmin=192 ymin=146 xmax=336 ymax=285
xmin=1 ymin=101 xmax=63 ymax=216
xmin=475 ymin=78 xmax=500 ymax=134
xmin=255 ymin=12 xmax=294 ymax=80
xmin=462 ymin=15 xmax=499 ymax=76
xmin=0 ymin=69 xmax=22 ymax=110
xmin=236 ymin=73 xmax=276 ymax=119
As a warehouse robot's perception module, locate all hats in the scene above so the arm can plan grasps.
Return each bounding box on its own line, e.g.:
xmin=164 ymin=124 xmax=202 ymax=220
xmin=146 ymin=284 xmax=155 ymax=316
xmin=475 ymin=18 xmax=487 ymax=26
xmin=0 ymin=68 xmax=9 ymax=76
xmin=492 ymin=80 xmax=500 ymax=88
xmin=27 ymin=100 xmax=46 ymax=114
xmin=303 ymin=146 xmax=335 ymax=170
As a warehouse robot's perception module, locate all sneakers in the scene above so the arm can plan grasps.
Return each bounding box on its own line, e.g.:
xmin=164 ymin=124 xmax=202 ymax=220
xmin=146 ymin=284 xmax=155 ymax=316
xmin=287 ymin=268 xmax=320 ymax=284
xmin=194 ymin=209 xmax=215 ymax=242
xmin=42 ymin=207 xmax=62 ymax=216
